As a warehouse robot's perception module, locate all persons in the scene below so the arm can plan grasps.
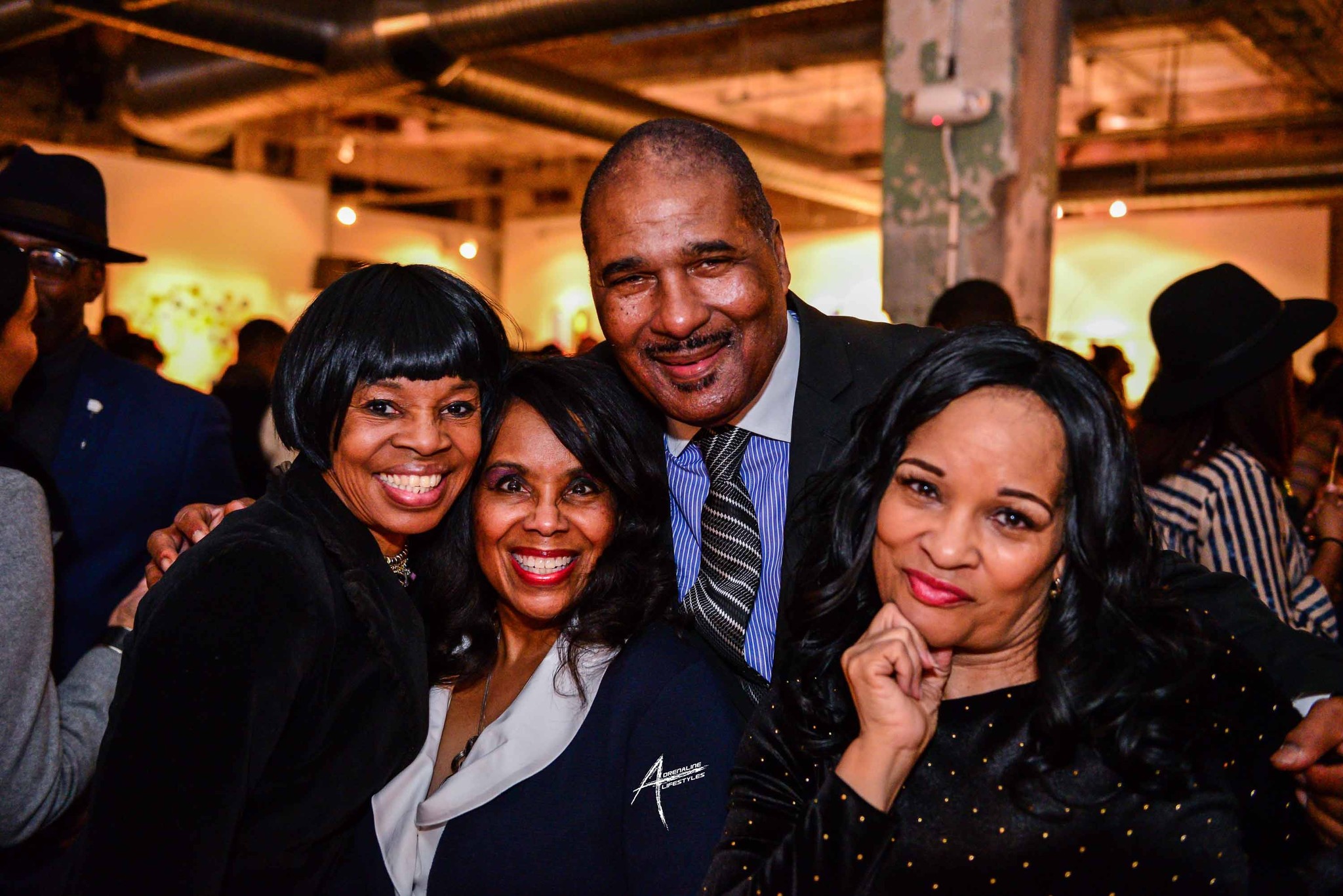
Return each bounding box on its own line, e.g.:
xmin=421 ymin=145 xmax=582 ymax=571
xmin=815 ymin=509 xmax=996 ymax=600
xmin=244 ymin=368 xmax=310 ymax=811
xmin=0 ymin=118 xmax=1343 ymax=896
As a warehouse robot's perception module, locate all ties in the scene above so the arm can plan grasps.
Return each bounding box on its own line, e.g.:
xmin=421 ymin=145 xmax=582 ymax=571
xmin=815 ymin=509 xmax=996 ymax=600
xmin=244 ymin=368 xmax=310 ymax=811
xmin=676 ymin=426 xmax=761 ymax=658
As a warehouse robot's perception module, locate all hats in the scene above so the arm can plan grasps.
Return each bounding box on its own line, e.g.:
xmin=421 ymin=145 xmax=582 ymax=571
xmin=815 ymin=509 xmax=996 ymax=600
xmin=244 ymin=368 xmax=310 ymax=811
xmin=1132 ymin=262 xmax=1339 ymax=419
xmin=0 ymin=146 xmax=147 ymax=263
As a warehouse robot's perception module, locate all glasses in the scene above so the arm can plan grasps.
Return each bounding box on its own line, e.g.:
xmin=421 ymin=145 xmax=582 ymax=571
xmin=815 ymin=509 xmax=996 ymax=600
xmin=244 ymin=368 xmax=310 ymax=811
xmin=21 ymin=243 xmax=100 ymax=285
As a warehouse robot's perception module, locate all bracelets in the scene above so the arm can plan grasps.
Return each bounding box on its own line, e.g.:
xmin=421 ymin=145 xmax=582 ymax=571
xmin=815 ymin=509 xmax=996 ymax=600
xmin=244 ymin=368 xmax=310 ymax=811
xmin=99 ymin=624 xmax=131 ymax=650
xmin=1315 ymin=536 xmax=1343 ymax=548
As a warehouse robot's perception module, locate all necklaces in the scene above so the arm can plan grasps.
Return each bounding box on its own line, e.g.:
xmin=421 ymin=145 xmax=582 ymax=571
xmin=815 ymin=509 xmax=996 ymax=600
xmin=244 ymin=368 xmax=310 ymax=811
xmin=451 ymin=672 xmax=491 ymax=775
